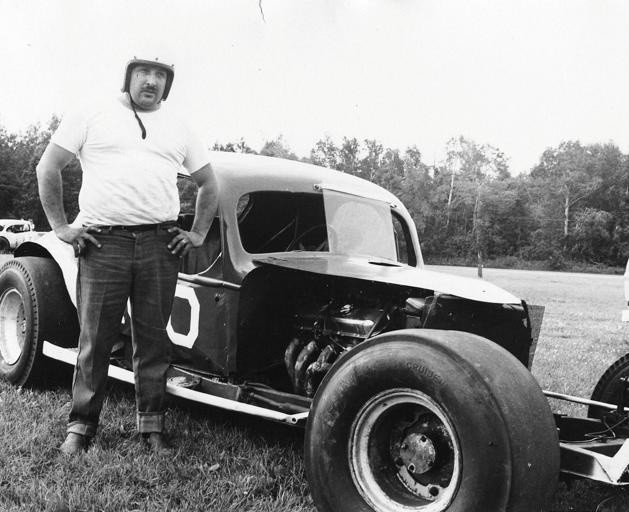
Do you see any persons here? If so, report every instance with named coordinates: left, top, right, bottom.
left=33, top=36, right=225, bottom=459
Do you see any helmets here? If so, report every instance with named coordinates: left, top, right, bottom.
left=120, top=55, right=175, bottom=101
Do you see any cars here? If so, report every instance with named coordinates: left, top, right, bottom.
left=0, top=153, right=629, bottom=512
left=1, top=219, right=39, bottom=252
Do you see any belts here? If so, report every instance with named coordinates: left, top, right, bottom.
left=96, top=221, right=176, bottom=231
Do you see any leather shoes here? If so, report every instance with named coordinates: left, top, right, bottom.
left=142, top=432, right=171, bottom=451
left=59, top=432, right=86, bottom=453
